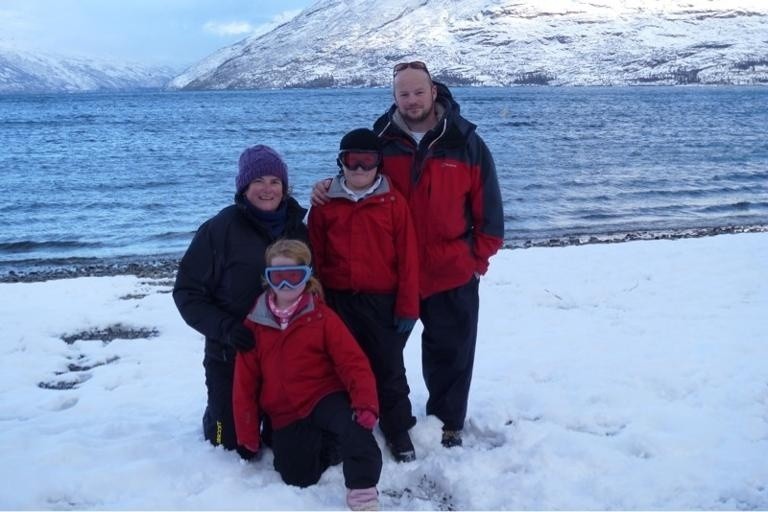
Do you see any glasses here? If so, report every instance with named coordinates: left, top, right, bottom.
left=265, top=263, right=313, bottom=289
left=392, top=61, right=426, bottom=71
left=338, top=149, right=382, bottom=171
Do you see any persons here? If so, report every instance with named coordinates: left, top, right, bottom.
left=172, top=144, right=310, bottom=451
left=232, top=239, right=383, bottom=511
left=307, top=128, right=422, bottom=463
left=309, top=61, right=504, bottom=447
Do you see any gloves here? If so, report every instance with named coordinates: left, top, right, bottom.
left=222, top=323, right=256, bottom=353
left=355, top=409, right=377, bottom=428
left=394, top=316, right=416, bottom=335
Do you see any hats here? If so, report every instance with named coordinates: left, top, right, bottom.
left=337, top=128, right=384, bottom=174
left=236, top=143, right=288, bottom=197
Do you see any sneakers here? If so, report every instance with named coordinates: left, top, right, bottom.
left=347, top=485, right=380, bottom=510
left=441, top=428, right=462, bottom=447
left=390, top=430, right=416, bottom=461
